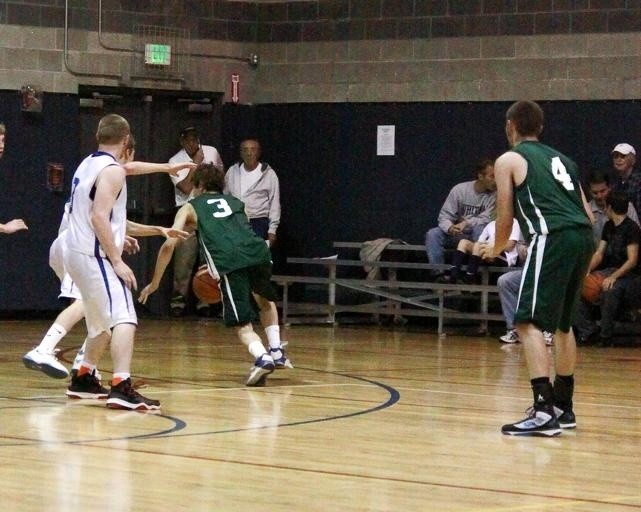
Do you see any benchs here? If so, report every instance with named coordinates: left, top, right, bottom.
left=262, top=235, right=527, bottom=339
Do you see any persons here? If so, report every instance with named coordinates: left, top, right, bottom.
left=425, top=161, right=497, bottom=284
left=477, top=95, right=598, bottom=436
left=573, top=189, right=641, bottom=348
left=0, top=120, right=30, bottom=235
left=166, top=124, right=229, bottom=319
left=497, top=242, right=554, bottom=345
left=439, top=219, right=524, bottom=284
left=588, top=169, right=640, bottom=250
left=223, top=134, right=284, bottom=252
left=612, top=143, right=640, bottom=221
left=138, top=161, right=294, bottom=386
left=63, top=112, right=161, bottom=410
left=22, top=131, right=199, bottom=383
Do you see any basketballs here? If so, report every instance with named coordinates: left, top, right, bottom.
left=581, top=271, right=607, bottom=302
left=193, top=266, right=220, bottom=305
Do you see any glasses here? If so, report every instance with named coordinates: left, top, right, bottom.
left=614, top=154, right=627, bottom=158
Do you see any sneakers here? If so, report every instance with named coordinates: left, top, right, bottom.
left=170, top=297, right=185, bottom=317
left=462, top=272, right=477, bottom=284
left=500, top=330, right=517, bottom=343
left=198, top=302, right=211, bottom=317
left=269, top=342, right=293, bottom=369
left=246, top=353, right=275, bottom=386
left=597, top=330, right=617, bottom=345
left=543, top=331, right=556, bottom=346
left=580, top=333, right=600, bottom=348
left=524, top=404, right=576, bottom=429
left=23, top=346, right=160, bottom=412
left=437, top=271, right=457, bottom=285
left=502, top=403, right=561, bottom=436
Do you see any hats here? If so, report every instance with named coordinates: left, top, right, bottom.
left=611, top=143, right=636, bottom=155
